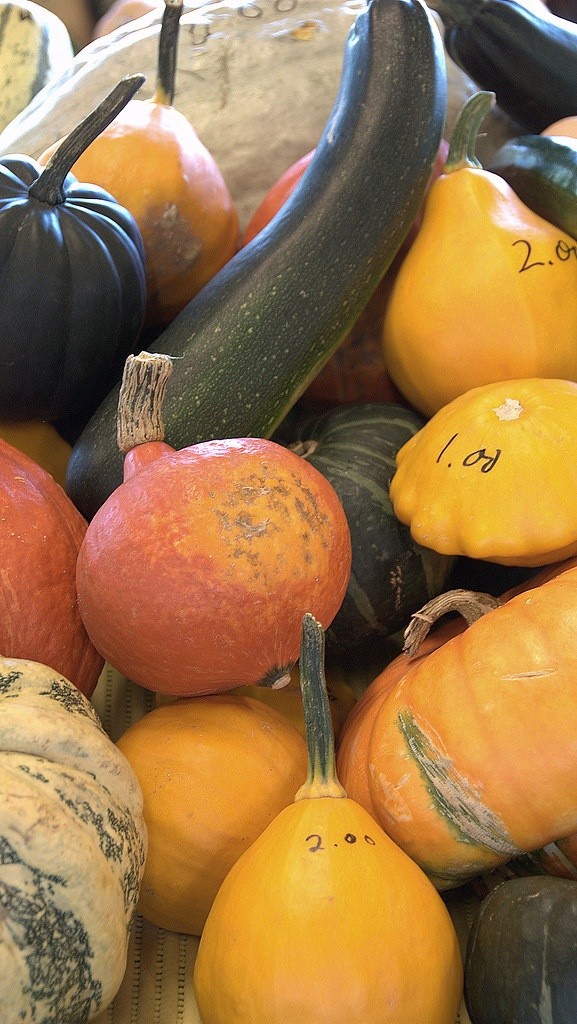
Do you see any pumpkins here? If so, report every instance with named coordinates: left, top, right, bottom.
left=0, top=0, right=577, bottom=1024
